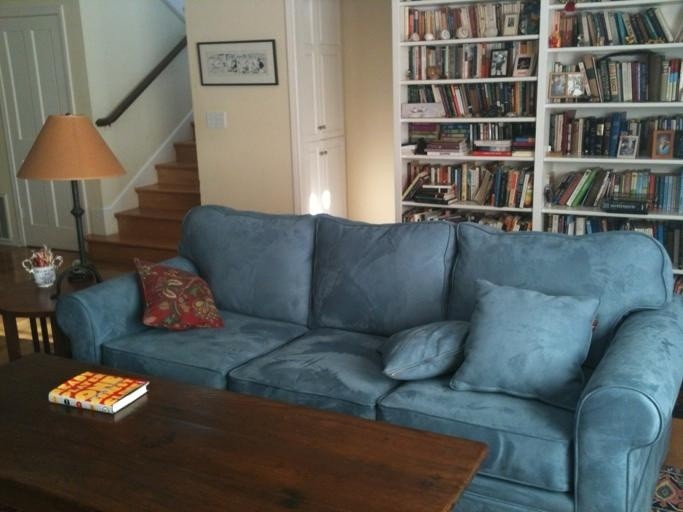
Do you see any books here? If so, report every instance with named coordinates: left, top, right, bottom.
left=48, top=371, right=150, bottom=414
left=48, top=394, right=149, bottom=423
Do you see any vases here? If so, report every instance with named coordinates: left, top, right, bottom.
left=19, top=256, right=62, bottom=287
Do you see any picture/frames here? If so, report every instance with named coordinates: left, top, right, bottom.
left=193, top=39, right=278, bottom=87
left=489, top=48, right=510, bottom=78
left=651, top=129, right=674, bottom=159
left=499, top=11, right=521, bottom=37
left=548, top=71, right=584, bottom=103
left=616, top=134, right=639, bottom=159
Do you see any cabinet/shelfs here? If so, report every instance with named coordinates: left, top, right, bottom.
left=545, top=2, right=682, bottom=274
left=285, top=0, right=346, bottom=220
left=393, top=1, right=541, bottom=234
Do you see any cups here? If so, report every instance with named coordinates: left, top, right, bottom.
left=31, top=266, right=55, bottom=289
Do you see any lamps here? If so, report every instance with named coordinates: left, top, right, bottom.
left=16, top=112, right=129, bottom=288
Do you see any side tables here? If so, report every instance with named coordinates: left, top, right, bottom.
left=0, top=254, right=100, bottom=366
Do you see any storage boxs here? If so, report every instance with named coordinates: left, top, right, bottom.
left=399, top=103, right=445, bottom=118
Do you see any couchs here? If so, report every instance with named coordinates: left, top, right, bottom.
left=55, top=204, right=682, bottom=511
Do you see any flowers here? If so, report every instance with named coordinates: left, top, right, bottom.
left=27, top=243, right=55, bottom=268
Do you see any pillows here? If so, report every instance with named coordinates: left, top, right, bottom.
left=446, top=274, right=601, bottom=413
left=377, top=319, right=471, bottom=383
left=132, top=259, right=223, bottom=332
